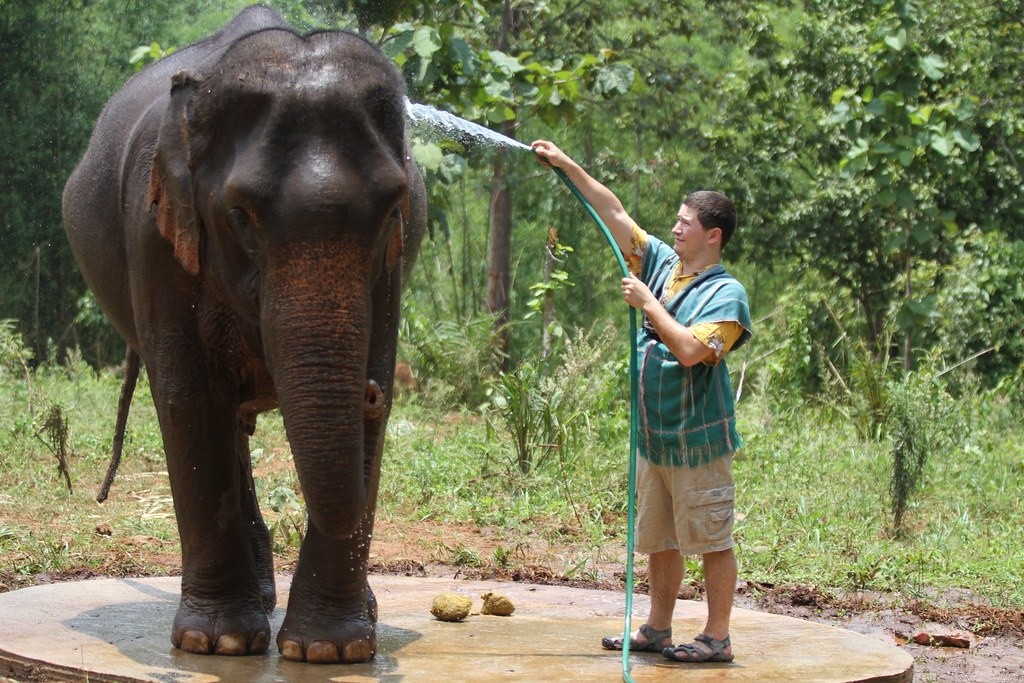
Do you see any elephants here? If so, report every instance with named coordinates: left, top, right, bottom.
left=61, top=2, right=429, bottom=666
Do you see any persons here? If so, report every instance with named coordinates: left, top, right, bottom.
left=531, top=139, right=753, bottom=663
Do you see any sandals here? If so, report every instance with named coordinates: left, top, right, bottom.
left=602, top=624, right=675, bottom=652
left=663, top=634, right=735, bottom=662
